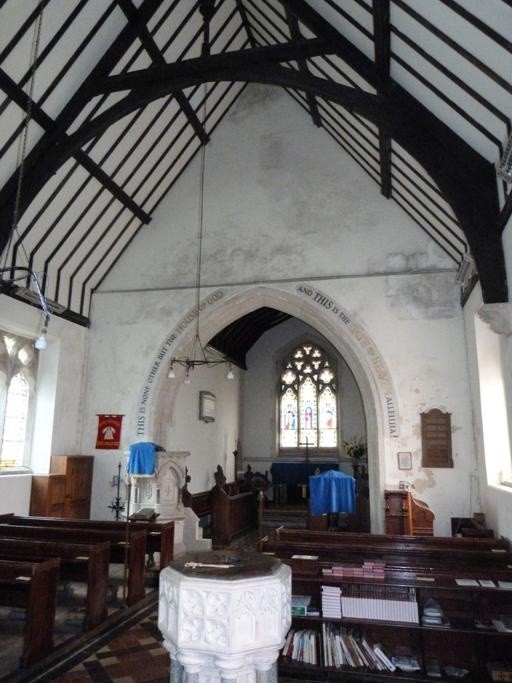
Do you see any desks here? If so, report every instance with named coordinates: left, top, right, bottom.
left=158, top=547, right=294, bottom=683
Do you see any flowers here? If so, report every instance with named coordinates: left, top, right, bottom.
left=345, top=438, right=365, bottom=458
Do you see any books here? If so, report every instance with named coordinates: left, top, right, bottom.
left=280, top=553, right=511, bottom=683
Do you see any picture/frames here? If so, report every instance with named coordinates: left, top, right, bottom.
left=398, top=452, right=412, bottom=470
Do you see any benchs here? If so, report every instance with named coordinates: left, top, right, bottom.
left=451, top=517, right=493, bottom=537
left=1, top=512, right=175, bottom=670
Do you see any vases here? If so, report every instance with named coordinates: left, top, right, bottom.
left=351, top=456, right=359, bottom=466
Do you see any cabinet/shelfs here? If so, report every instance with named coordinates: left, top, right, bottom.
left=51, top=455, right=94, bottom=519
left=257, top=526, right=512, bottom=682
left=272, top=462, right=339, bottom=505
left=30, top=475, right=68, bottom=517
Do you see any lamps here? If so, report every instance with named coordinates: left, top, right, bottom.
left=168, top=82, right=234, bottom=385
left=1, top=11, right=52, bottom=351
